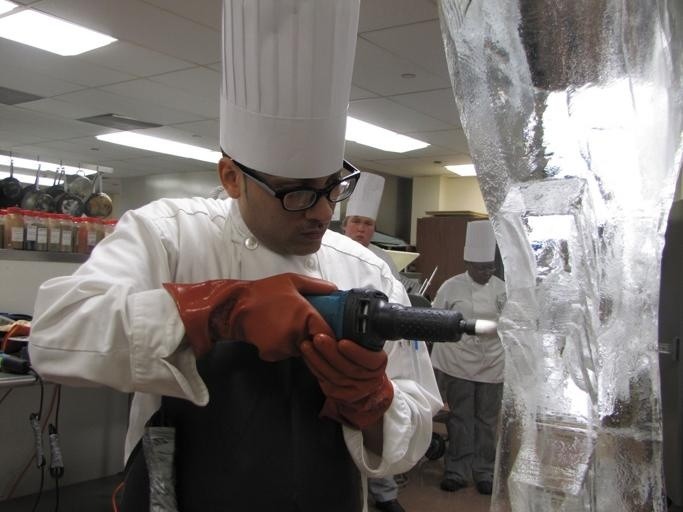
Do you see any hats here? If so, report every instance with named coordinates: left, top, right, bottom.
left=346, top=172, right=385, bottom=221
left=464, top=220, right=496, bottom=263
left=220, top=0, right=360, bottom=179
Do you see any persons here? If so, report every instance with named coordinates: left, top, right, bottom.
left=27, top=147, right=445, bottom=509
left=429, top=260, right=507, bottom=495
left=339, top=215, right=407, bottom=511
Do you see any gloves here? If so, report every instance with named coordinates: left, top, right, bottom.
left=163, top=272, right=336, bottom=363
left=297, top=333, right=394, bottom=431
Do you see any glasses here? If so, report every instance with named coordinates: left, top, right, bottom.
left=230, top=159, right=361, bottom=212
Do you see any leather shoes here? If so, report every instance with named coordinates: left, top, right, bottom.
left=375, top=498, right=406, bottom=511
left=440, top=479, right=494, bottom=493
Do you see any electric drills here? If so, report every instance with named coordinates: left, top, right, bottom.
left=306, top=283, right=567, bottom=352
